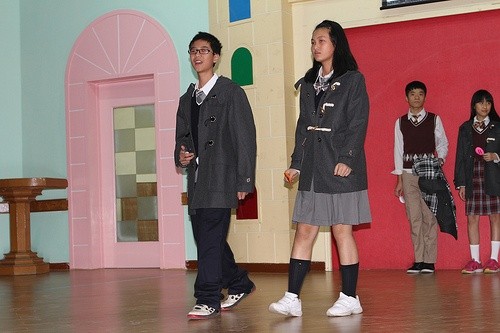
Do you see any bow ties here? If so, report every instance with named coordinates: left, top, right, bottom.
left=474, top=121, right=485, bottom=129
left=313, top=76, right=329, bottom=95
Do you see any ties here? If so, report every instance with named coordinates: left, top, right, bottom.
left=412, top=114, right=421, bottom=125
left=196, top=91, right=206, bottom=103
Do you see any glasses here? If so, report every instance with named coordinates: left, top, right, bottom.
left=188, top=48, right=215, bottom=55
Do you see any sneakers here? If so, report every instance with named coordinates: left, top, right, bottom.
left=327, top=291, right=363, bottom=317
left=484, top=259, right=500, bottom=273
left=221, top=284, right=256, bottom=311
left=406, top=262, right=424, bottom=273
left=420, top=263, right=435, bottom=273
left=461, top=260, right=483, bottom=274
left=269, top=291, right=302, bottom=317
left=187, top=303, right=221, bottom=320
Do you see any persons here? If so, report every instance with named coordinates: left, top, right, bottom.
left=391, top=81, right=448, bottom=273
left=453, top=89, right=500, bottom=274
left=174, top=32, right=257, bottom=320
left=269, top=20, right=372, bottom=317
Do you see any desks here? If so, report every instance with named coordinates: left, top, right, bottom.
left=0, top=177, right=68, bottom=276
